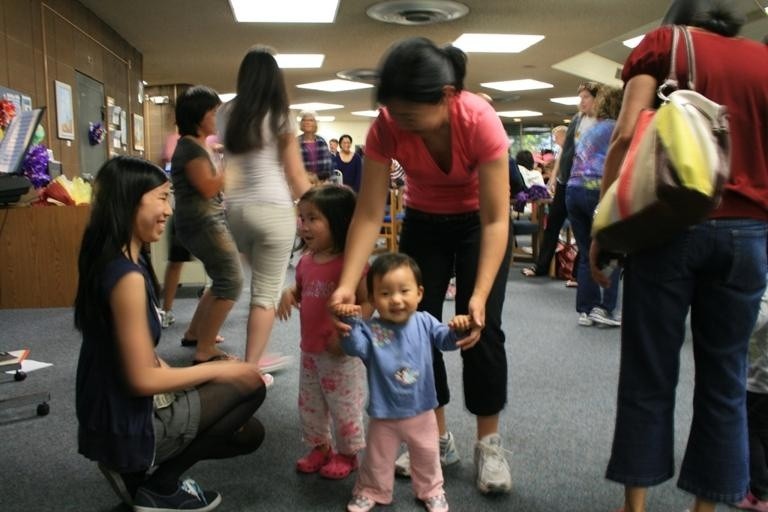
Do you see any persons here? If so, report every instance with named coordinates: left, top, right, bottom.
left=322, top=34, right=513, bottom=497
left=587, top=1, right=766, bottom=511
left=158, top=127, right=222, bottom=328
left=293, top=113, right=330, bottom=176
left=570, top=90, right=626, bottom=329
left=73, top=158, right=268, bottom=509
left=386, top=160, right=407, bottom=206
left=212, top=46, right=317, bottom=386
left=737, top=291, right=766, bottom=510
left=171, top=86, right=243, bottom=368
left=275, top=184, right=374, bottom=479
left=329, top=135, right=363, bottom=190
left=477, top=84, right=600, bottom=287
left=332, top=255, right=475, bottom=510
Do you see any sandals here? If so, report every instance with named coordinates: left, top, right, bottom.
left=294, top=442, right=360, bottom=480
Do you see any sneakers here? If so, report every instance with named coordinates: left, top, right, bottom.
left=347, top=492, right=376, bottom=512
left=577, top=305, right=622, bottom=328
left=161, top=310, right=176, bottom=330
left=256, top=355, right=294, bottom=387
left=472, top=431, right=513, bottom=495
left=394, top=431, right=461, bottom=477
left=423, top=494, right=449, bottom=512
left=520, top=245, right=534, bottom=256
left=92, top=456, right=223, bottom=512
left=734, top=490, right=768, bottom=511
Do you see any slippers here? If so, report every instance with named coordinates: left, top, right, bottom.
left=564, top=275, right=581, bottom=287
left=522, top=265, right=537, bottom=278
left=179, top=334, right=241, bottom=365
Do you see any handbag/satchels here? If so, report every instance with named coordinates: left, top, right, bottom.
left=589, top=22, right=732, bottom=262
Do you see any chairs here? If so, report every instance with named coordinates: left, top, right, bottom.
left=374, top=181, right=565, bottom=282
